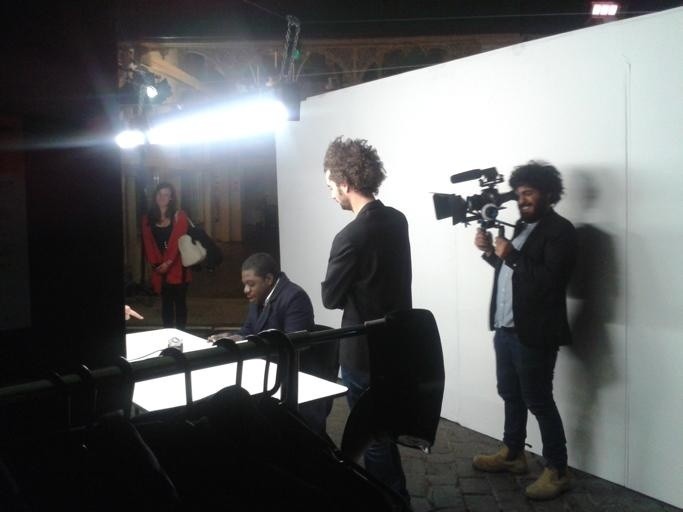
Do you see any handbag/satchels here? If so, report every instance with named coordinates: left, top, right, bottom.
left=178, top=234, right=207, bottom=267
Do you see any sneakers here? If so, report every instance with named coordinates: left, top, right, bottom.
left=521, top=466, right=576, bottom=500
left=472, top=444, right=528, bottom=474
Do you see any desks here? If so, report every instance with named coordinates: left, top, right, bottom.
left=121, top=325, right=347, bottom=429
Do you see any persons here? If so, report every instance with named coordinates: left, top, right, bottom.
left=319, top=135, right=413, bottom=509
left=206, top=253, right=315, bottom=370
left=470, top=161, right=577, bottom=503
left=123, top=302, right=144, bottom=323
left=142, top=179, right=192, bottom=333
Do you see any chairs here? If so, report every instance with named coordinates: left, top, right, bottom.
left=304, top=325, right=342, bottom=436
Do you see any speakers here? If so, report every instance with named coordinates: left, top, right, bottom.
left=24, top=141, right=126, bottom=360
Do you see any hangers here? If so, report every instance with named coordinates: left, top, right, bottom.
left=49, top=323, right=299, bottom=463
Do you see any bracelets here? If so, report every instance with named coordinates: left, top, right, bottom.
left=166, top=260, right=172, bottom=267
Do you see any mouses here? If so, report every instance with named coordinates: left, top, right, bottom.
left=159, top=348, right=181, bottom=356
